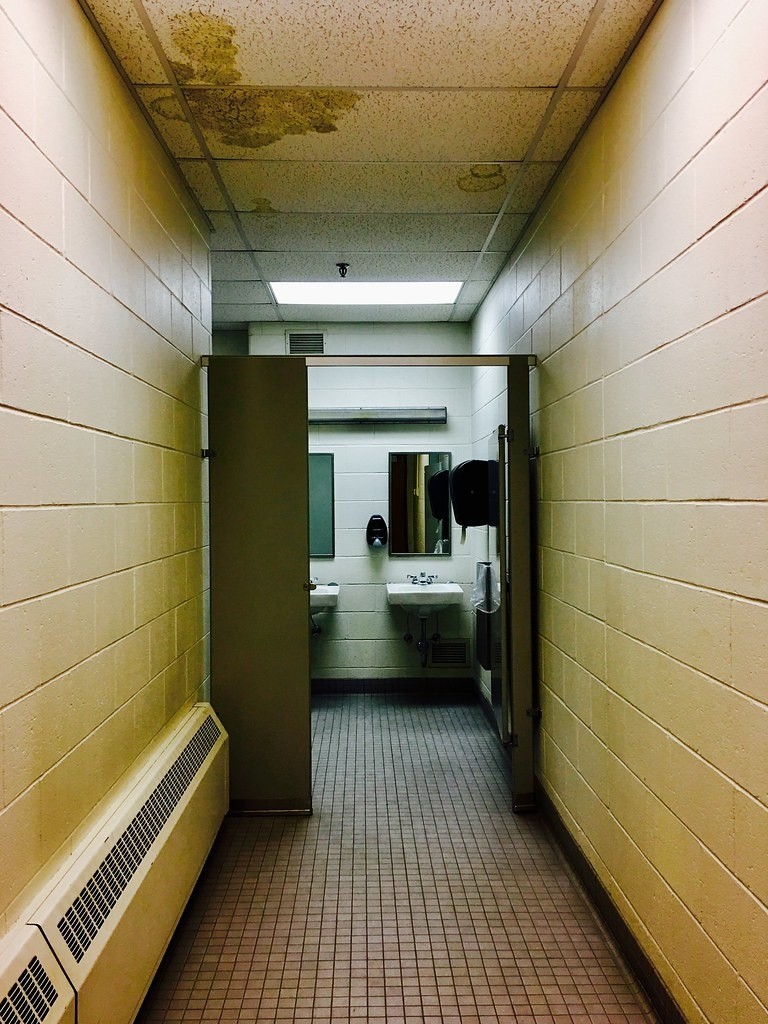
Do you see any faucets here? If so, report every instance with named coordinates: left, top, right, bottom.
left=419, top=571, right=427, bottom=584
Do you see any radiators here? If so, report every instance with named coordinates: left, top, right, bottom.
left=0, top=700, right=231, bottom=1021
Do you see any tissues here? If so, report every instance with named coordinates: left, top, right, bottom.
left=448, top=458, right=500, bottom=546
left=425, top=469, right=449, bottom=535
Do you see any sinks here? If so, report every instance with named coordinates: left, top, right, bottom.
left=386, top=582, right=464, bottom=618
left=309, top=585, right=340, bottom=616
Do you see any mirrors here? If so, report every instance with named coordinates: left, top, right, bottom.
left=389, top=450, right=452, bottom=557
left=308, top=452, right=336, bottom=558
